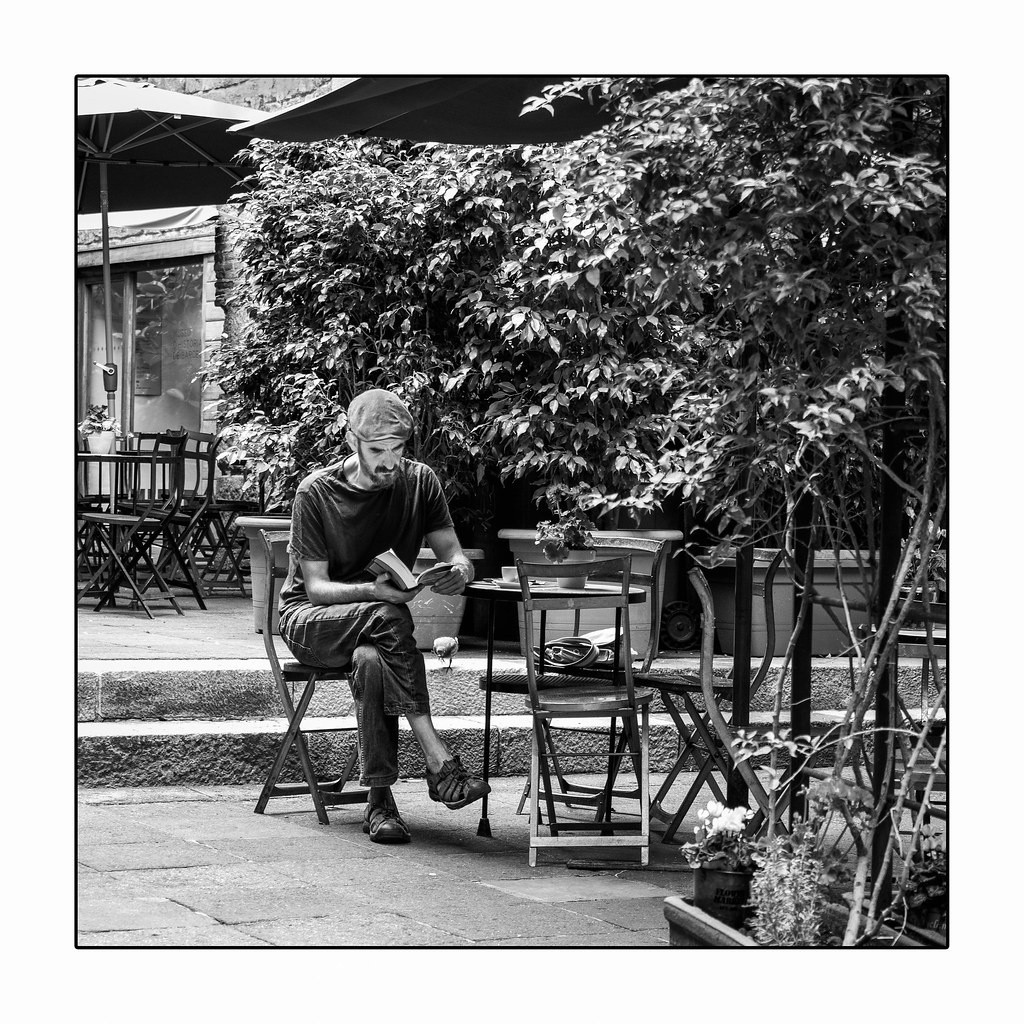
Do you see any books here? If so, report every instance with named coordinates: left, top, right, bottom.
left=365, top=548, right=456, bottom=592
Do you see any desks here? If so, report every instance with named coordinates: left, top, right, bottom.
left=856, top=623, right=949, bottom=843
left=460, top=580, right=647, bottom=837
left=77, top=452, right=181, bottom=608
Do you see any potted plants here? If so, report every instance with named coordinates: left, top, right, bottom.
left=498, top=529, right=684, bottom=661
left=403, top=548, right=485, bottom=651
left=77, top=403, right=120, bottom=454
left=214, top=420, right=301, bottom=635
left=534, top=508, right=598, bottom=589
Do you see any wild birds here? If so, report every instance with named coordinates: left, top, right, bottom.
left=432, top=636, right=459, bottom=676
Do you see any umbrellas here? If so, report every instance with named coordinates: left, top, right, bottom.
left=222, top=75, right=730, bottom=152
left=74, top=75, right=337, bottom=608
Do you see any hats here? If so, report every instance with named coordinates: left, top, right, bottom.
left=348, top=388, right=414, bottom=443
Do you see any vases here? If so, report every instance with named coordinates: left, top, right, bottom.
left=694, top=868, right=758, bottom=929
left=664, top=895, right=925, bottom=947
left=702, top=547, right=880, bottom=657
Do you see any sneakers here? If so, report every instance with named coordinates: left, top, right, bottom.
left=360, top=793, right=410, bottom=842
left=425, top=755, right=492, bottom=810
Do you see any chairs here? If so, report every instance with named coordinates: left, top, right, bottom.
left=630, top=547, right=789, bottom=844
left=254, top=529, right=370, bottom=826
left=75, top=425, right=265, bottom=619
left=516, top=531, right=670, bottom=823
left=515, top=554, right=653, bottom=867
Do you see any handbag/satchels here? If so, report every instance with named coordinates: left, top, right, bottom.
left=531, top=626, right=637, bottom=670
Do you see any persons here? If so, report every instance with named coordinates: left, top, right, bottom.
left=276, top=385, right=490, bottom=842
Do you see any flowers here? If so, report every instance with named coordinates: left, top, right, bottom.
left=678, top=800, right=755, bottom=869
